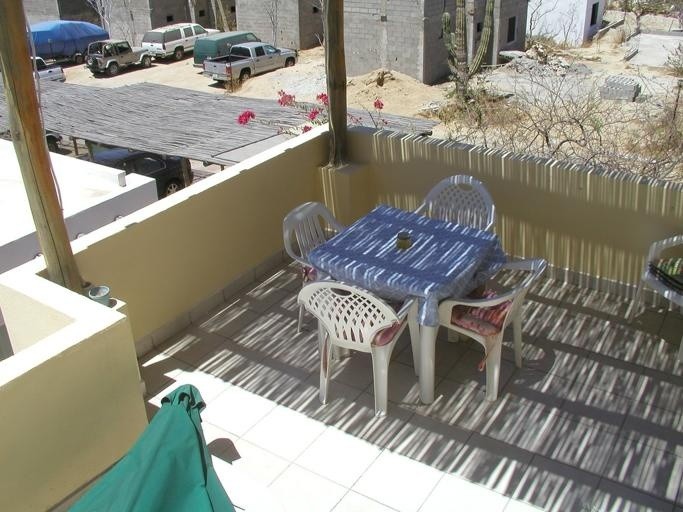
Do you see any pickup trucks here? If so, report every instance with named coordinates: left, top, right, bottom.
left=204, top=42, right=297, bottom=84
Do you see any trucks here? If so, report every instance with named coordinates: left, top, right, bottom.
left=29, top=56, right=66, bottom=84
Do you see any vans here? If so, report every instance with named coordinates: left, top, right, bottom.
left=24, top=20, right=110, bottom=64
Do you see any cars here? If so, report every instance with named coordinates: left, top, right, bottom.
left=80, top=149, right=193, bottom=199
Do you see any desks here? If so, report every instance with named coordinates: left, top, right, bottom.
left=310, top=205, right=490, bottom=405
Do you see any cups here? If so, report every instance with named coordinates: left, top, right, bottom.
left=88, top=285, right=110, bottom=306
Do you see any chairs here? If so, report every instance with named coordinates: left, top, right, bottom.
left=298, top=281, right=422, bottom=419
left=625, top=237, right=683, bottom=363
left=439, top=258, right=547, bottom=404
left=283, top=201, right=344, bottom=332
left=414, top=176, right=496, bottom=338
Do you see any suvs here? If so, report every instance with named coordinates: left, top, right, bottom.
left=87, top=40, right=151, bottom=76
left=191, top=31, right=261, bottom=68
left=143, top=23, right=220, bottom=61
